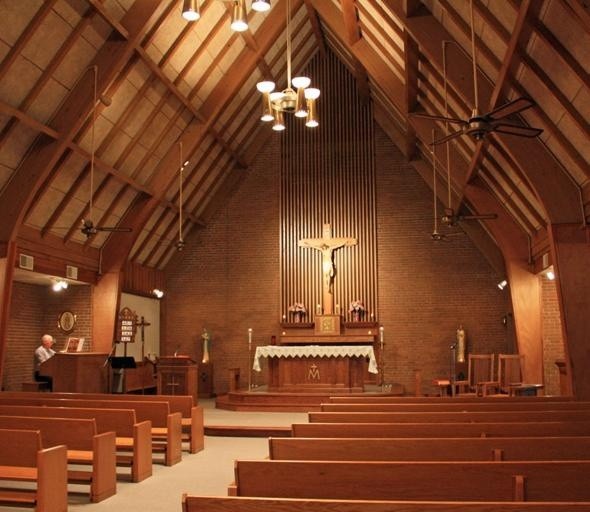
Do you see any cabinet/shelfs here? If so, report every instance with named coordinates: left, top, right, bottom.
left=159, top=365, right=197, bottom=406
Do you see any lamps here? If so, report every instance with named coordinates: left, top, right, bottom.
left=256, top=0, right=321, bottom=130
left=183, top=0, right=271, bottom=31
left=498, top=280, right=507, bottom=290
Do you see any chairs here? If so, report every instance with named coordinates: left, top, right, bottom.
left=455, top=353, right=495, bottom=397
left=484, top=354, right=526, bottom=397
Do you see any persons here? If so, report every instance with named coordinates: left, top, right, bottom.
left=299, top=240, right=354, bottom=292
left=456, top=324, right=466, bottom=363
left=74, top=340, right=79, bottom=351
left=70, top=340, right=74, bottom=351
left=34, top=334, right=56, bottom=392
left=201, top=327, right=210, bottom=363
left=64, top=312, right=71, bottom=328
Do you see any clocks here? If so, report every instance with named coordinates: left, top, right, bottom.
left=58, top=310, right=77, bottom=334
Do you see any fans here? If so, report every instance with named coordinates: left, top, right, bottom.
left=430, top=128, right=464, bottom=242
left=177, top=140, right=187, bottom=251
left=442, top=42, right=497, bottom=235
left=46, top=66, right=132, bottom=233
left=408, top=0, right=543, bottom=145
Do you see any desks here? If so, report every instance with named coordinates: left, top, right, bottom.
left=252, top=345, right=378, bottom=392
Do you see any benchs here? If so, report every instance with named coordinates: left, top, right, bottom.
left=308, top=409, right=590, bottom=423
left=1, top=428, right=68, bottom=512
left=0, top=415, right=117, bottom=502
left=181, top=493, right=590, bottom=512
left=227, top=459, right=590, bottom=502
left=0, top=404, right=152, bottom=482
left=23, top=381, right=48, bottom=391
left=292, top=421, right=590, bottom=438
left=0, top=390, right=204, bottom=454
left=330, top=397, right=590, bottom=403
left=269, top=436, right=590, bottom=462
left=321, top=403, right=590, bottom=412
left=0, top=396, right=181, bottom=466
